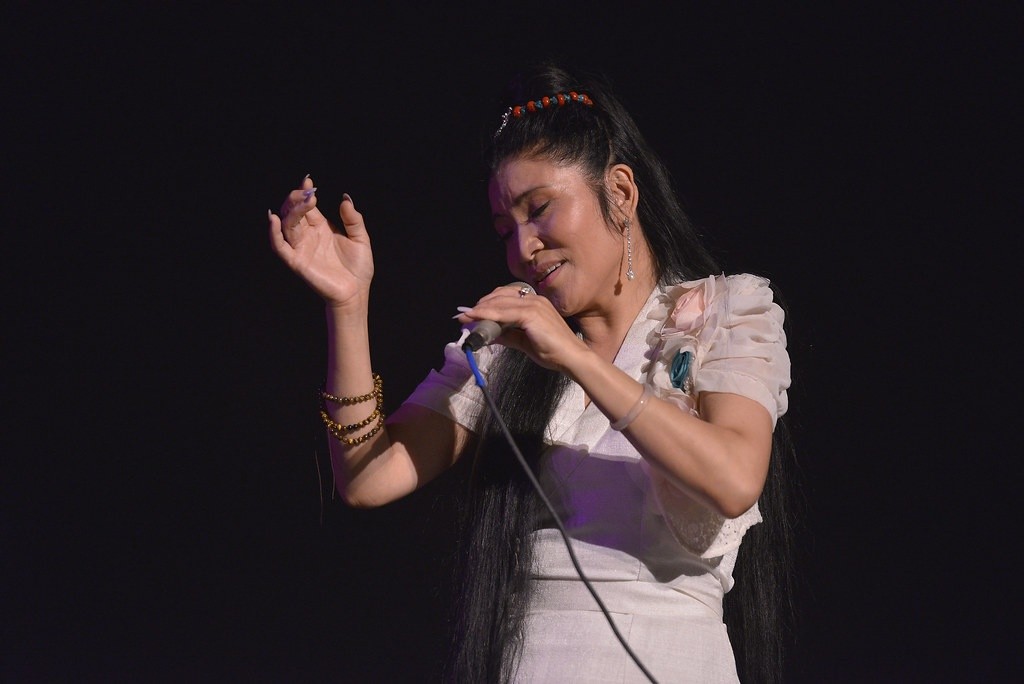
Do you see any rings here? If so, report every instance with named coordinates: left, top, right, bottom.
left=519, top=284, right=530, bottom=296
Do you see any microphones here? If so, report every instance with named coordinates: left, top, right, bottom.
left=461, top=281, right=537, bottom=352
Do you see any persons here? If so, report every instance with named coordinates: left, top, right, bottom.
left=267, top=56, right=801, bottom=684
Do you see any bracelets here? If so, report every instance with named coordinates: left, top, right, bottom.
left=611, top=382, right=650, bottom=430
left=318, top=374, right=385, bottom=443
